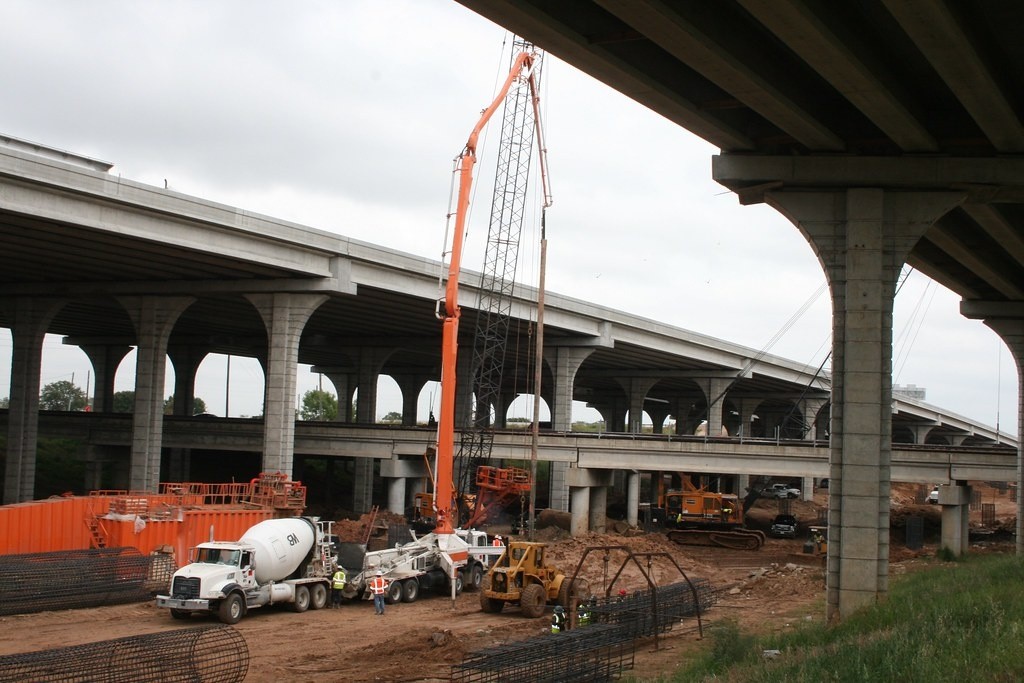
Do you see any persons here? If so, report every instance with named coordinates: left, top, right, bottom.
left=551, top=605, right=567, bottom=634
left=578, top=595, right=598, bottom=628
left=370, top=571, right=388, bottom=615
left=492, top=535, right=504, bottom=564
left=616, top=590, right=628, bottom=603
left=721, top=504, right=729, bottom=522
left=332, top=565, right=346, bottom=609
left=813, top=531, right=824, bottom=551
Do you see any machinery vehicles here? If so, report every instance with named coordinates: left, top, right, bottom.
left=657, top=470, right=765, bottom=550
left=336, top=33, right=589, bottom=618
left=156, top=515, right=331, bottom=625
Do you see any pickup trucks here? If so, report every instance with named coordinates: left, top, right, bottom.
left=929, top=485, right=938, bottom=503
left=760, top=483, right=800, bottom=500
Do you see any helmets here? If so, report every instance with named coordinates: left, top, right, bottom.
left=498, top=536, right=502, bottom=539
left=554, top=606, right=564, bottom=613
left=495, top=534, right=498, bottom=538
left=589, top=596, right=597, bottom=601
left=376, top=571, right=382, bottom=575
left=579, top=605, right=585, bottom=610
left=337, top=565, right=342, bottom=569
left=618, top=589, right=626, bottom=595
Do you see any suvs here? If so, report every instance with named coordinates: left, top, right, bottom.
left=770, top=515, right=800, bottom=538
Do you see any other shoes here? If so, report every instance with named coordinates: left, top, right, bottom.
left=375, top=612, right=379, bottom=615
left=379, top=613, right=384, bottom=615
